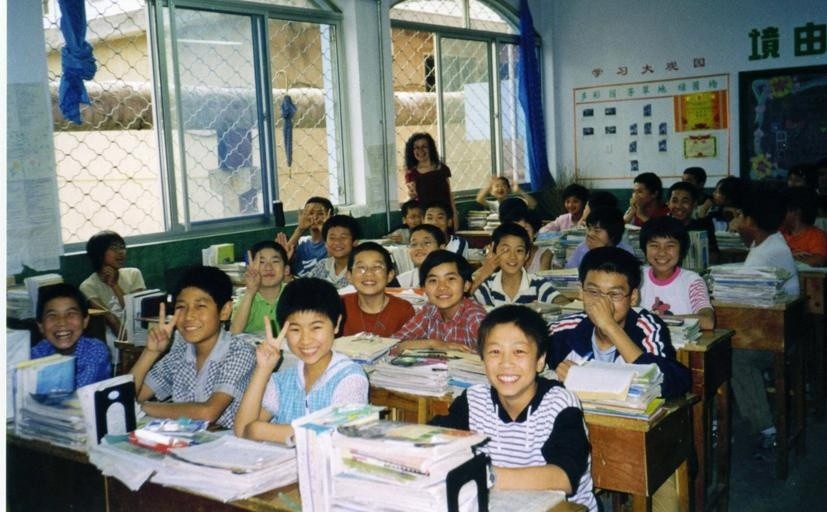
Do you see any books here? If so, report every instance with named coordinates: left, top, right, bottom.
left=6, top=283, right=34, bottom=321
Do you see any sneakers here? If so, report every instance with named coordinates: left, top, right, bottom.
left=713, top=427, right=735, bottom=448
left=745, top=433, right=776, bottom=464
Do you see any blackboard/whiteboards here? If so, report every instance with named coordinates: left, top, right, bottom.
left=738, top=63, right=827, bottom=184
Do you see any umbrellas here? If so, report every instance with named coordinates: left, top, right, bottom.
left=274, top=70, right=296, bottom=178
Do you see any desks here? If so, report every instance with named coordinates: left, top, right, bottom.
left=793, top=254, right=827, bottom=457
left=9, top=308, right=704, bottom=512
left=709, top=292, right=816, bottom=475
left=674, top=323, right=739, bottom=511
left=453, top=231, right=540, bottom=275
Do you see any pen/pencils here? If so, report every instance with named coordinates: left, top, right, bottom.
left=579, top=351, right=593, bottom=365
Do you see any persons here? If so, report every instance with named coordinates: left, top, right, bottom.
left=29, top=134, right=826, bottom=512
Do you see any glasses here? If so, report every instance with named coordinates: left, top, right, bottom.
left=583, top=287, right=629, bottom=301
